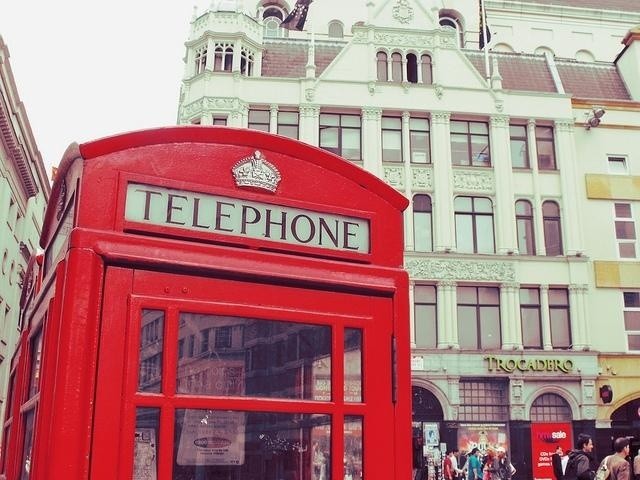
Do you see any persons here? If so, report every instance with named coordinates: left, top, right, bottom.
left=426, top=430, right=437, bottom=443
left=442, top=447, right=516, bottom=480
left=551, top=445, right=563, bottom=480
left=633, top=449, right=640, bottom=479
left=562, top=433, right=596, bottom=480
left=594, top=437, right=630, bottom=480
left=562, top=449, right=573, bottom=474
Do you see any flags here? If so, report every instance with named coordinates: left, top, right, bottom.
left=476, top=7, right=492, bottom=49
left=284, top=3, right=310, bottom=34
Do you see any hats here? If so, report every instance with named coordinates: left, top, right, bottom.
left=495, top=447, right=506, bottom=453
left=615, top=438, right=630, bottom=449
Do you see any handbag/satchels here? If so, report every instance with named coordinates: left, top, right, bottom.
left=594, top=465, right=609, bottom=480
left=510, top=463, right=516, bottom=475
